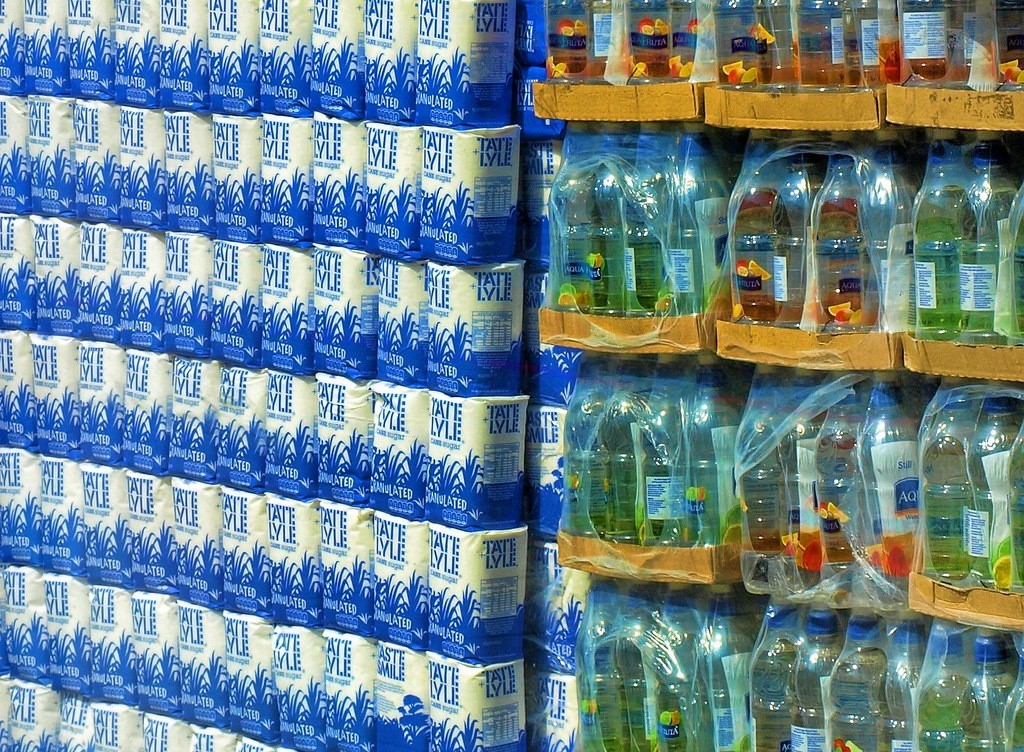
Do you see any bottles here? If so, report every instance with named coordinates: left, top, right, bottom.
left=562, top=354, right=1024, bottom=752
left=551, top=121, right=1024, bottom=345
left=544, top=0, right=1024, bottom=95
